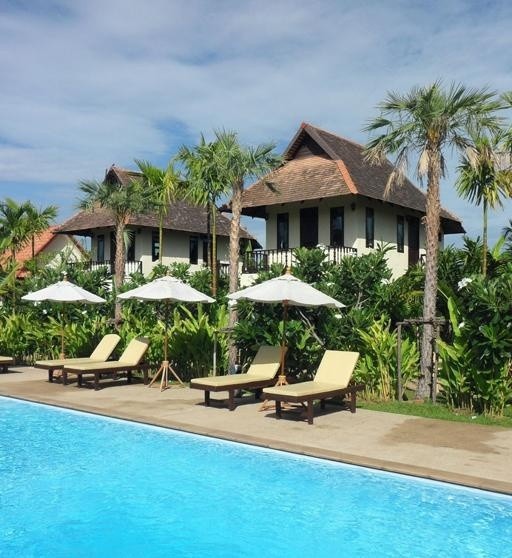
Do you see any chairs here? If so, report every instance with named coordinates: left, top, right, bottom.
left=190, top=344, right=366, bottom=425
left=35, top=333, right=152, bottom=391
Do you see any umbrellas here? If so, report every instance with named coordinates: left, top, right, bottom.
left=20, top=276, right=108, bottom=353
left=115, top=275, right=218, bottom=360
left=225, top=271, right=348, bottom=376
